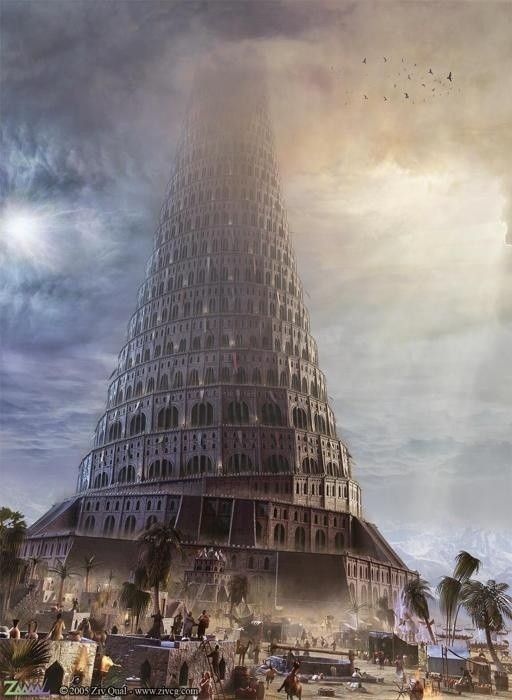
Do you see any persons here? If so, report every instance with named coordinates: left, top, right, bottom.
left=264, top=665, right=277, bottom=690
left=206, top=645, right=222, bottom=676
left=348, top=649, right=404, bottom=688
left=200, top=671, right=213, bottom=700
left=410, top=681, right=423, bottom=700
left=9, top=619, right=21, bottom=639
left=44, top=614, right=66, bottom=641
left=145, top=606, right=210, bottom=640
left=459, top=667, right=472, bottom=686
left=295, top=629, right=336, bottom=656
